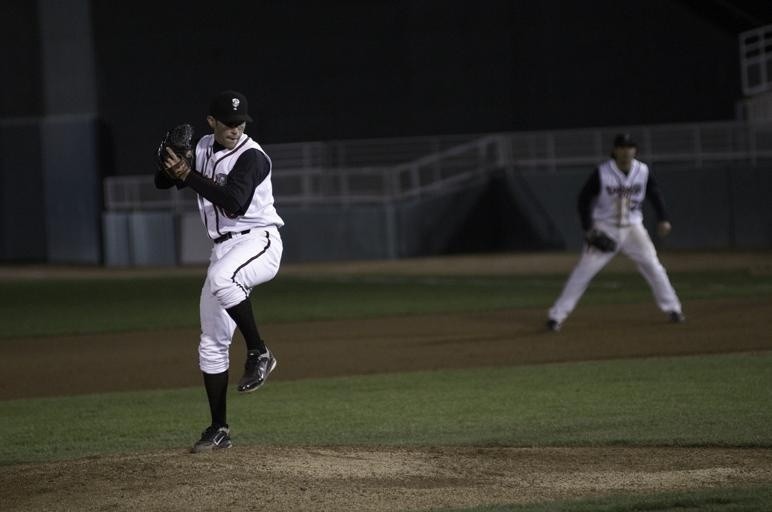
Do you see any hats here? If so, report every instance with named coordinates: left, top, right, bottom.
left=614, top=137, right=637, bottom=146
left=209, top=91, right=253, bottom=123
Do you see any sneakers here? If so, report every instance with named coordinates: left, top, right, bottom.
left=192, top=425, right=232, bottom=453
left=239, top=346, right=277, bottom=393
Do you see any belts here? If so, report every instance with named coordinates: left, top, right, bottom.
left=213, top=229, right=250, bottom=243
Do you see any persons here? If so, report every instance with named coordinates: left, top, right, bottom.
left=544, top=134, right=685, bottom=328
left=155, top=92, right=285, bottom=453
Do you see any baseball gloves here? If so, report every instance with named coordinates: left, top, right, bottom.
left=155, top=124, right=193, bottom=170
left=594, top=231, right=616, bottom=253
